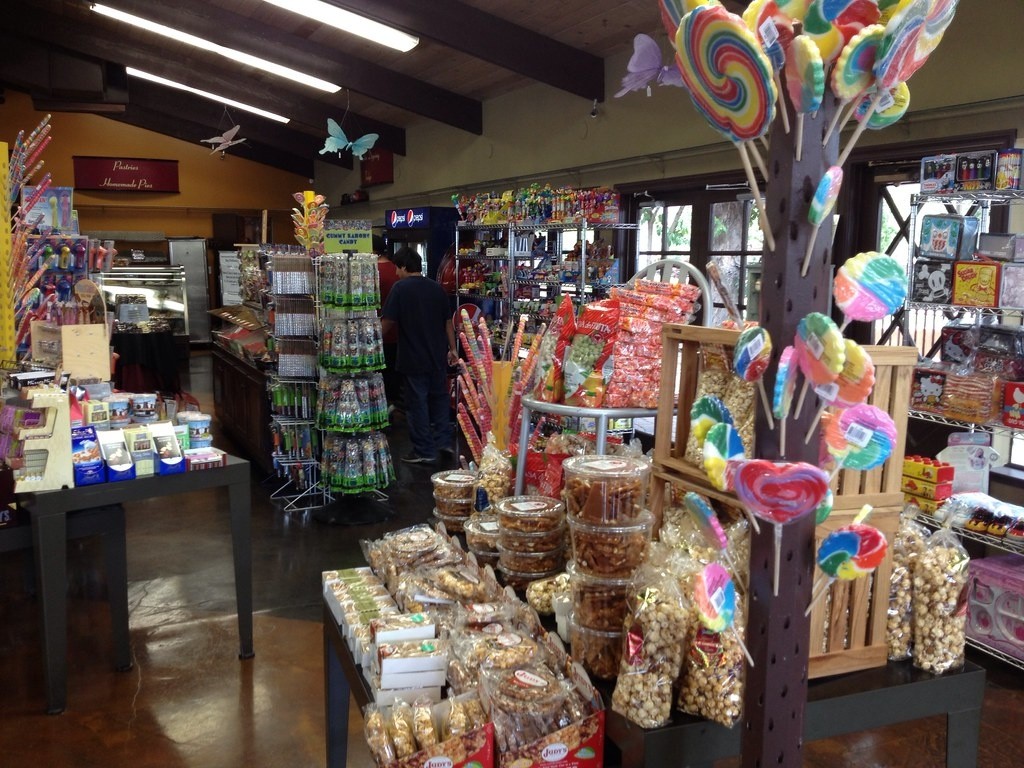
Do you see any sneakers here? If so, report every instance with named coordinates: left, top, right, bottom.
left=438, top=447, right=454, bottom=457
left=400, top=448, right=438, bottom=465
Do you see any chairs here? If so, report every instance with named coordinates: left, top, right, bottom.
left=513, top=259, right=711, bottom=496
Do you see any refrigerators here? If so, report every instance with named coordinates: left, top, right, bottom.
left=383, top=205, right=457, bottom=278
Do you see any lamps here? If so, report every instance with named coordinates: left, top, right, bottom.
left=84, top=0, right=420, bottom=124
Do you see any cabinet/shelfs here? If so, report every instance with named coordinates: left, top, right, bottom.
left=456, top=221, right=640, bottom=455
left=100, top=245, right=395, bottom=525
left=901, top=190, right=1024, bottom=670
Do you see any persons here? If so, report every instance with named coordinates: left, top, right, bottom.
left=380, top=247, right=459, bottom=463
left=372, top=234, right=399, bottom=416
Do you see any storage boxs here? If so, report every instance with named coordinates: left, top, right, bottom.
left=71, top=393, right=228, bottom=486
left=901, top=147, right=1024, bottom=661
left=322, top=521, right=606, bottom=768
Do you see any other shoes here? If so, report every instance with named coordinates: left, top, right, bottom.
left=387, top=404, right=395, bottom=415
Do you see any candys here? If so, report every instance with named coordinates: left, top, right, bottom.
left=455, top=306, right=547, bottom=462
left=655, top=0, right=960, bottom=666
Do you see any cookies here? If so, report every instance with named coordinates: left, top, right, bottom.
left=324, top=444, right=651, bottom=765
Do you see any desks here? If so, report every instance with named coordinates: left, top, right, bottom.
left=324, top=600, right=376, bottom=768
left=0, top=446, right=256, bottom=715
left=427, top=516, right=988, bottom=768
left=0, top=502, right=135, bottom=672
left=110, top=332, right=182, bottom=399
